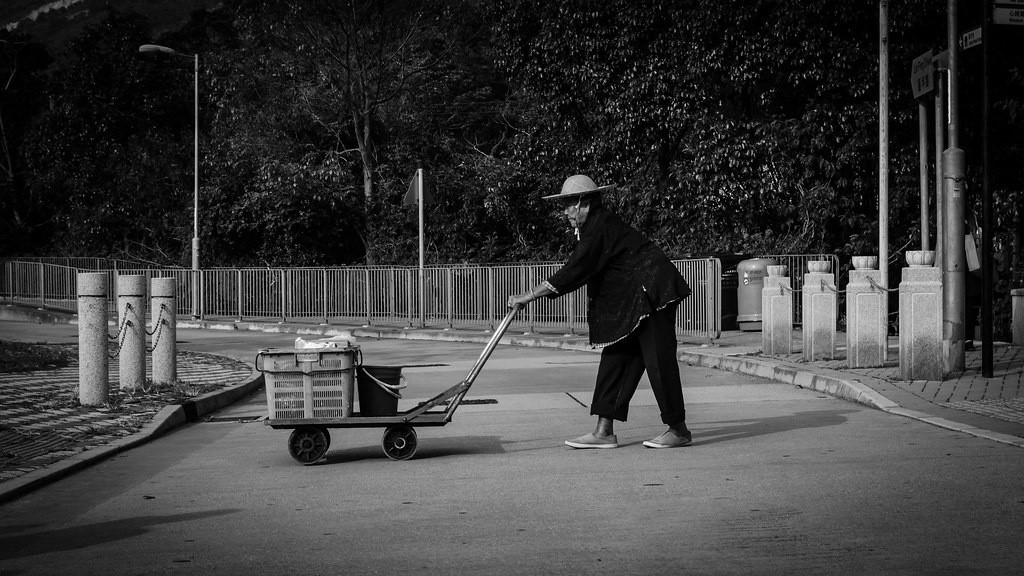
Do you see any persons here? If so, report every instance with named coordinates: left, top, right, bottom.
left=507, top=174, right=693, bottom=449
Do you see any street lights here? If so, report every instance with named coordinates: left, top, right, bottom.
left=138, top=42, right=202, bottom=319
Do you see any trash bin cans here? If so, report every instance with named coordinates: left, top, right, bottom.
left=736, top=258, right=774, bottom=332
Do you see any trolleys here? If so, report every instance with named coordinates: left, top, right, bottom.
left=238, top=301, right=524, bottom=465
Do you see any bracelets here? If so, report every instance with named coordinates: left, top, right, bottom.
left=529, top=287, right=537, bottom=301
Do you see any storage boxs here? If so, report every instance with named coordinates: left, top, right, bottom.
left=257, top=345, right=362, bottom=417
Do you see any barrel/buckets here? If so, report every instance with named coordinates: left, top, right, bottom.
left=355, top=365, right=407, bottom=416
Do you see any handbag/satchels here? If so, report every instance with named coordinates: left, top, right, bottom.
left=965, top=214, right=983, bottom=271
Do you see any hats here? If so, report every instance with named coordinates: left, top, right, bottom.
left=541, top=174, right=617, bottom=199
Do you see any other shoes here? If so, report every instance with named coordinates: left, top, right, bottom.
left=643, top=428, right=692, bottom=448
left=966, top=341, right=976, bottom=352
left=564, top=433, right=619, bottom=448
